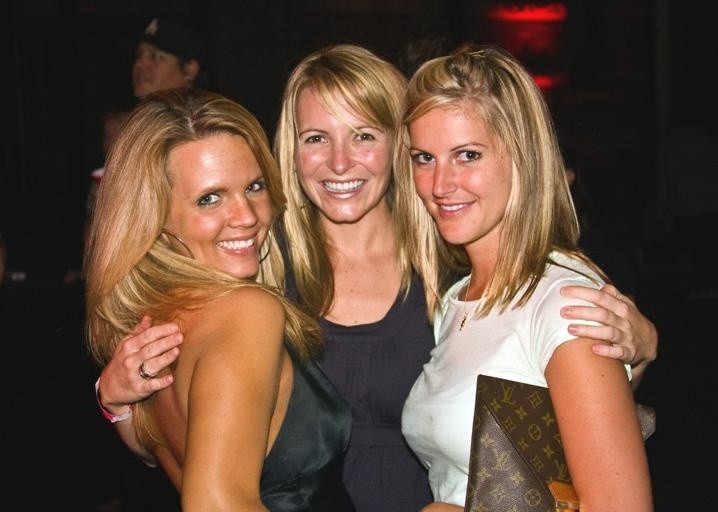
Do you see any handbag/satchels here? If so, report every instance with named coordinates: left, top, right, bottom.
left=464, top=374, right=655, bottom=512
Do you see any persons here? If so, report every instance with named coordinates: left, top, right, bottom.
left=397, top=44, right=661, bottom=510
left=78, top=41, right=660, bottom=511
left=75, top=9, right=208, bottom=207
left=75, top=87, right=362, bottom=512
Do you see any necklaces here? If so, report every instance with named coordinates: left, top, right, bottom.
left=458, top=267, right=496, bottom=330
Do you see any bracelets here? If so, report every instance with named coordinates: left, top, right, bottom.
left=94, top=378, right=135, bottom=423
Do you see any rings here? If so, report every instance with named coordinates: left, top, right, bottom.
left=138, top=362, right=155, bottom=379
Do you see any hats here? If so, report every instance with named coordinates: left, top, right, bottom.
left=143, top=9, right=209, bottom=64
